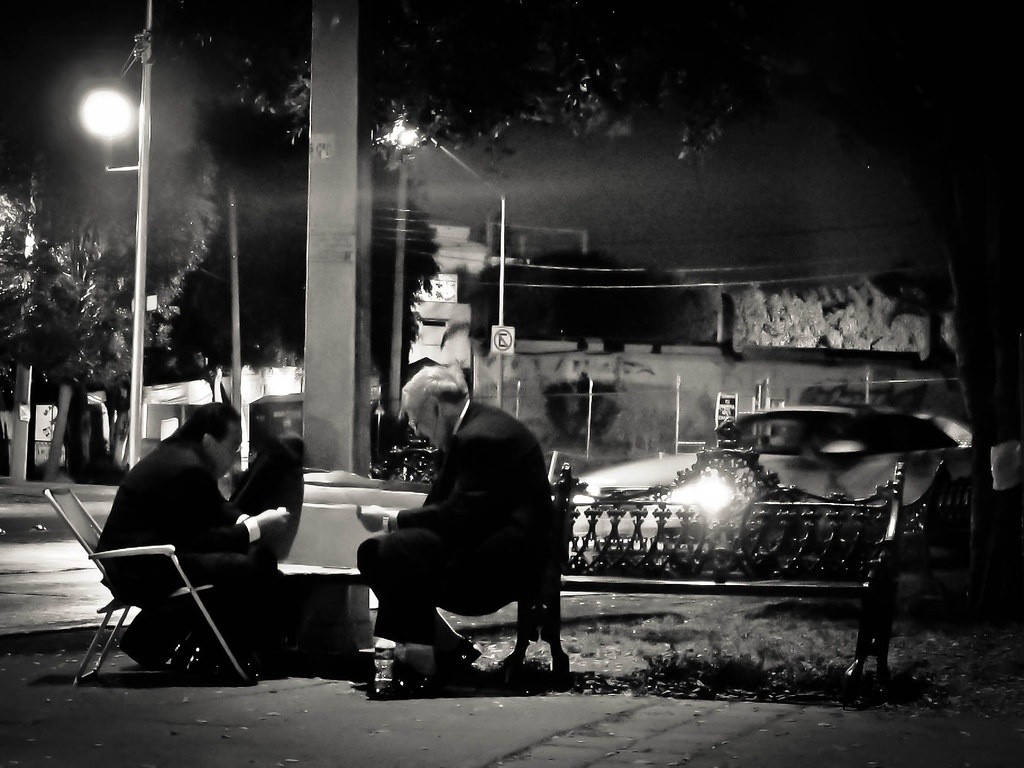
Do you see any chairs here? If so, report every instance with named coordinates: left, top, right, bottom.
left=44, top=486, right=257, bottom=686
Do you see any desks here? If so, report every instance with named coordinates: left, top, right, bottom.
left=274, top=562, right=369, bottom=670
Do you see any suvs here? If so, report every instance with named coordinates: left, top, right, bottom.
left=712, top=402, right=975, bottom=515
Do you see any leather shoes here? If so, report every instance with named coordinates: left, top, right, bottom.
left=365, top=659, right=436, bottom=701
left=444, top=638, right=485, bottom=681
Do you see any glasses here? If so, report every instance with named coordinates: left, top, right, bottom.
left=223, top=443, right=241, bottom=456
left=408, top=397, right=429, bottom=432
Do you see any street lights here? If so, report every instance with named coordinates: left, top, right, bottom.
left=385, top=118, right=506, bottom=410
left=77, top=89, right=153, bottom=467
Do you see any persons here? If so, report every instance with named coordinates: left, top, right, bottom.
left=356, top=366, right=554, bottom=697
left=95, top=403, right=289, bottom=671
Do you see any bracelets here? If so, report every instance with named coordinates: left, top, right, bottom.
left=382, top=513, right=390, bottom=528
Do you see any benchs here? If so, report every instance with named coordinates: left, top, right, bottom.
left=499, top=415, right=907, bottom=708
